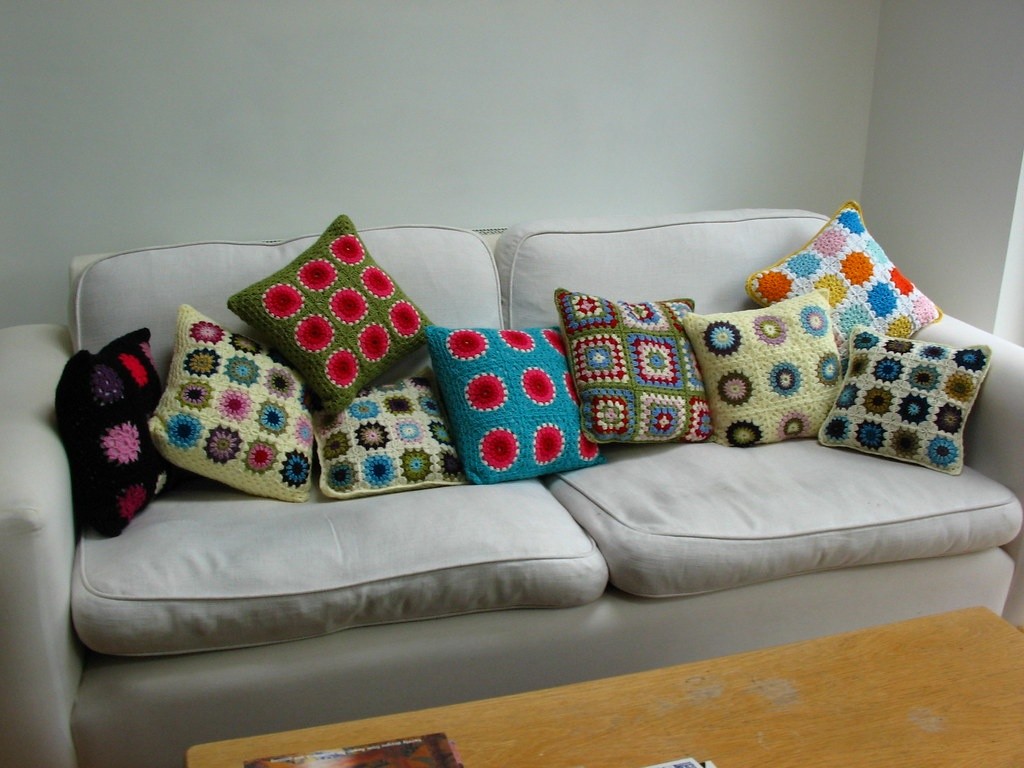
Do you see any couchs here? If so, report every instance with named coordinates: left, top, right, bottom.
left=0, top=208, right=1024, bottom=767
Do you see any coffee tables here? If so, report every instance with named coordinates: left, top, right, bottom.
left=185, top=604, right=1024, bottom=768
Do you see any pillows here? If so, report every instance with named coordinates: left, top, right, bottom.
left=147, top=303, right=315, bottom=503
left=817, top=326, right=992, bottom=476
left=53, top=326, right=180, bottom=536
left=679, top=289, right=848, bottom=448
left=745, top=200, right=944, bottom=375
left=226, top=213, right=437, bottom=419
left=307, top=364, right=468, bottom=498
left=425, top=321, right=606, bottom=485
left=550, top=283, right=712, bottom=444
